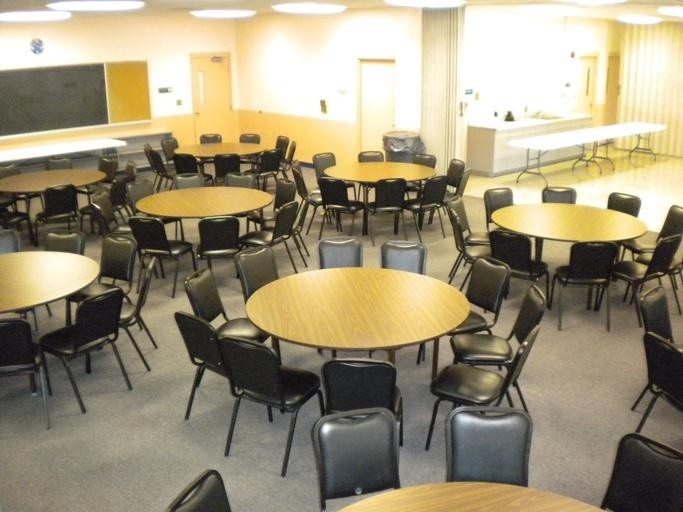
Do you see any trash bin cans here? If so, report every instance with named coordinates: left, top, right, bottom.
left=383, top=130, right=420, bottom=163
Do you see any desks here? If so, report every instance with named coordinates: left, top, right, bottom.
left=238, top=267, right=470, bottom=405
left=325, top=163, right=439, bottom=218
left=0, top=250, right=99, bottom=397
left=333, top=480, right=608, bottom=511
left=487, top=202, right=648, bottom=311
left=506, top=119, right=667, bottom=193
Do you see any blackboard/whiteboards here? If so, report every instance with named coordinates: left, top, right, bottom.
left=0, top=62, right=110, bottom=140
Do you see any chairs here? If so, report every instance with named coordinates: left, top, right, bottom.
left=613, top=234, right=682, bottom=323
left=218, top=334, right=321, bottom=477
left=185, top=268, right=283, bottom=365
left=441, top=404, right=536, bottom=489
left=489, top=230, right=549, bottom=309
left=542, top=185, right=576, bottom=206
left=1, top=228, right=21, bottom=253
left=416, top=256, right=511, bottom=365
left=607, top=192, right=642, bottom=218
left=550, top=242, right=617, bottom=332
left=40, top=287, right=133, bottom=408
left=484, top=187, right=513, bottom=228
left=234, top=246, right=280, bottom=303
left=263, top=196, right=321, bottom=267
left=1, top=314, right=53, bottom=430
left=0, top=130, right=466, bottom=243
left=446, top=198, right=491, bottom=253
left=317, top=237, right=362, bottom=268
left=425, top=327, right=542, bottom=452
left=629, top=332, right=683, bottom=433
left=94, top=257, right=158, bottom=373
left=640, top=285, right=683, bottom=350
left=240, top=201, right=298, bottom=276
left=447, top=209, right=492, bottom=289
left=642, top=205, right=682, bottom=313
left=323, top=359, right=404, bottom=446
left=449, top=285, right=547, bottom=370
left=173, top=311, right=275, bottom=423
left=45, top=231, right=85, bottom=254
left=597, top=430, right=683, bottom=511
left=127, top=213, right=197, bottom=297
left=196, top=216, right=241, bottom=280
left=310, top=406, right=404, bottom=512
left=379, top=241, right=427, bottom=274
left=65, top=234, right=143, bottom=330
left=162, top=468, right=234, bottom=512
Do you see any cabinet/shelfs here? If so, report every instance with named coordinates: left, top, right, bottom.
left=468, top=113, right=592, bottom=179
